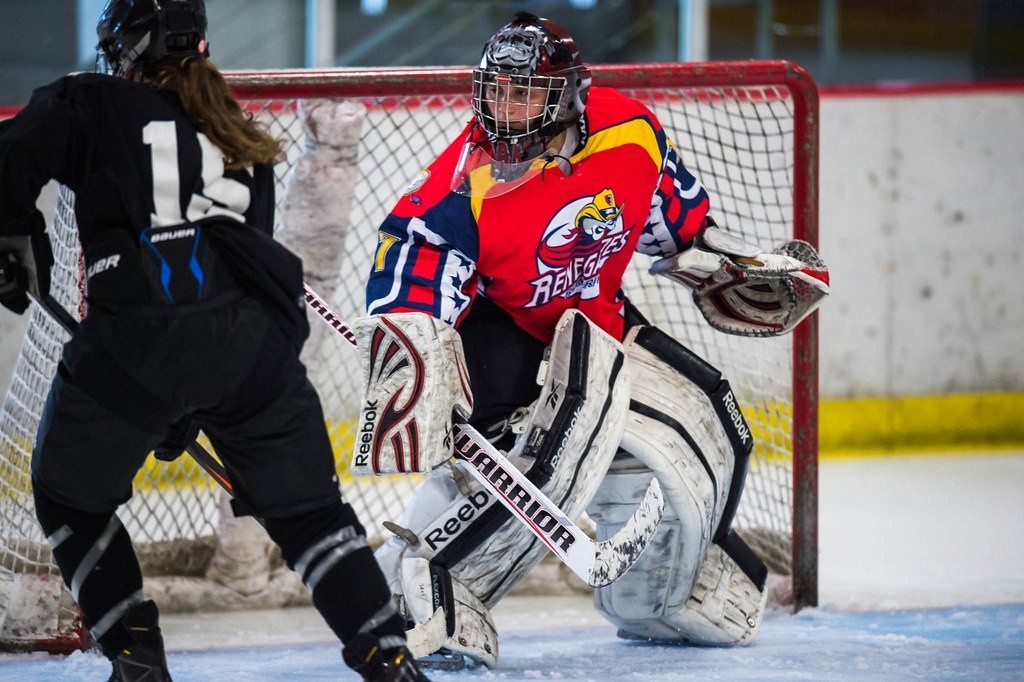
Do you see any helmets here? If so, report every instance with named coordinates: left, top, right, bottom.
left=93, top=0, right=209, bottom=81
left=471, top=10, right=593, bottom=182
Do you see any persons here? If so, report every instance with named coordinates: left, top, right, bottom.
left=0, top=0, right=433, bottom=682
left=350, top=11, right=830, bottom=670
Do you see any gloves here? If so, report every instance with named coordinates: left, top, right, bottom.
left=352, top=311, right=472, bottom=475
left=0, top=208, right=54, bottom=314
left=646, top=225, right=831, bottom=336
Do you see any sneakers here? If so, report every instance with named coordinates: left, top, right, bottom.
left=103, top=598, right=172, bottom=682
left=341, top=632, right=429, bottom=682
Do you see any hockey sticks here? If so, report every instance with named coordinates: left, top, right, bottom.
left=298, top=275, right=666, bottom=592
left=0, top=255, right=449, bottom=662
